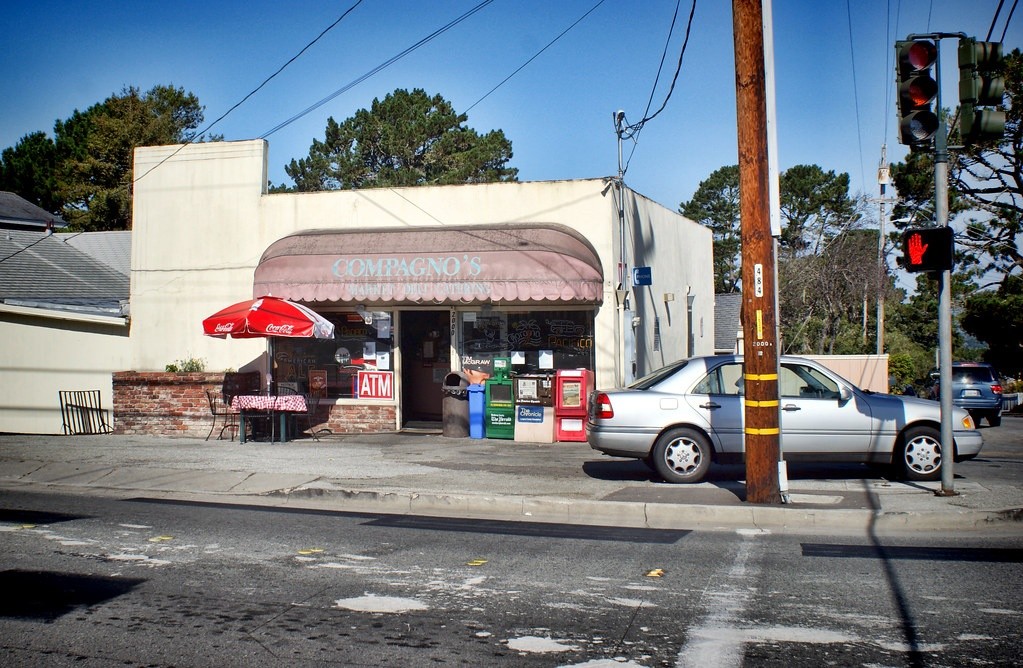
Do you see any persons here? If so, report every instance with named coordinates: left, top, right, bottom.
left=901, top=380, right=941, bottom=401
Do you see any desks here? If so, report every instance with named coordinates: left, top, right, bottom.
left=230, top=393, right=307, bottom=444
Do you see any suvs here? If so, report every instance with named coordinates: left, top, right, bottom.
left=925, top=360, right=1005, bottom=428
left=438, top=339, right=518, bottom=372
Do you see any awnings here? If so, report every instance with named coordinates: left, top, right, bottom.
left=253, top=222, right=604, bottom=302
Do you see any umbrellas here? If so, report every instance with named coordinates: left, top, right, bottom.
left=203, top=297, right=335, bottom=420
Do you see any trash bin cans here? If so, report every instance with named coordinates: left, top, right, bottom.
left=441, top=371, right=470, bottom=438
left=467, top=383, right=485, bottom=439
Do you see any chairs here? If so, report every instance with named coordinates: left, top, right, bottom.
left=205, top=384, right=325, bottom=443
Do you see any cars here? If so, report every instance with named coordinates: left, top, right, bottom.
left=589, top=353, right=985, bottom=484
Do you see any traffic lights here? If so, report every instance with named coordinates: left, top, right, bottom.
left=958, top=38, right=1008, bottom=147
left=897, top=38, right=940, bottom=146
left=898, top=226, right=956, bottom=274
